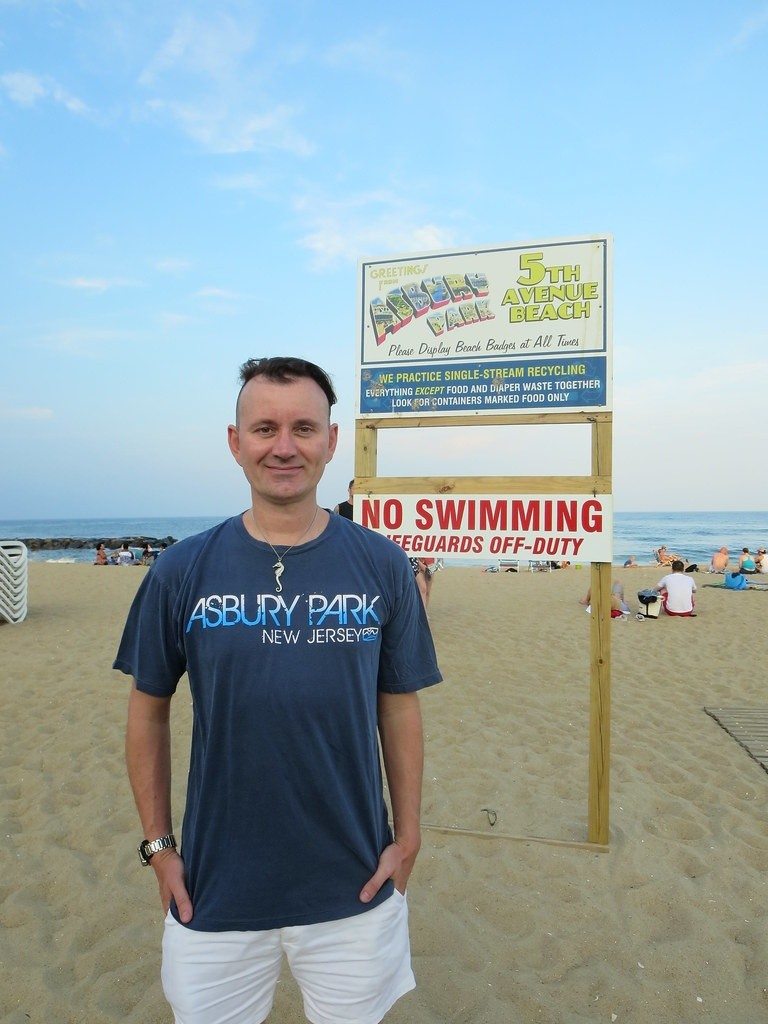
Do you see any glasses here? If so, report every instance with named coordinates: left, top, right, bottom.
left=662, top=547, right=666, bottom=549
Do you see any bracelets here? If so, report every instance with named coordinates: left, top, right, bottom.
left=137, top=834, right=177, bottom=866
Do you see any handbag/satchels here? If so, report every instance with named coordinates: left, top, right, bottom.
left=637, top=589, right=665, bottom=619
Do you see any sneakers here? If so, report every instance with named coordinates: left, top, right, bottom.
left=635, top=613, right=645, bottom=622
left=615, top=614, right=627, bottom=622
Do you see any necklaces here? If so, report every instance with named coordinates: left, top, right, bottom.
left=251, top=507, right=317, bottom=592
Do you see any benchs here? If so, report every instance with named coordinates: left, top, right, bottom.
left=528, top=561, right=551, bottom=573
left=498, top=559, right=520, bottom=572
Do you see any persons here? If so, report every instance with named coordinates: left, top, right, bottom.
left=411, top=558, right=443, bottom=609
left=579, top=580, right=631, bottom=615
left=333, top=480, right=354, bottom=521
left=112, top=358, right=441, bottom=1024
left=709, top=547, right=729, bottom=574
left=547, top=561, right=570, bottom=569
left=654, top=561, right=697, bottom=617
left=738, top=548, right=756, bottom=574
left=754, top=547, right=768, bottom=573
left=658, top=546, right=682, bottom=566
left=624, top=557, right=634, bottom=568
left=96, top=543, right=167, bottom=565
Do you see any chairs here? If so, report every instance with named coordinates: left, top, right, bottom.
left=653, top=550, right=673, bottom=567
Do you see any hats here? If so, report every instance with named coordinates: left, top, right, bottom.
left=757, top=546, right=767, bottom=552
